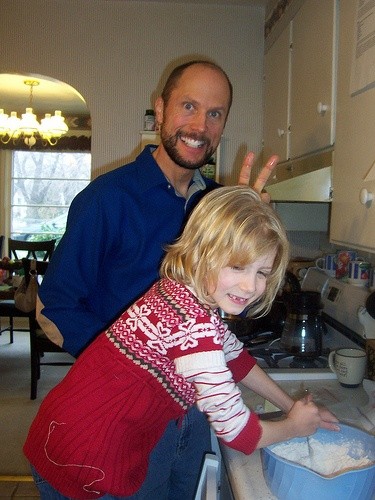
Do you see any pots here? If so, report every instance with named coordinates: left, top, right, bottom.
left=272, top=271, right=301, bottom=313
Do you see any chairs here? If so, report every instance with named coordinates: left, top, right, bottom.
left=0, top=236, right=74, bottom=400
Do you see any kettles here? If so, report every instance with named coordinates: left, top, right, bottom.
left=277, top=289, right=328, bottom=357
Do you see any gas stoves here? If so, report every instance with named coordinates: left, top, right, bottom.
left=221, top=267, right=375, bottom=380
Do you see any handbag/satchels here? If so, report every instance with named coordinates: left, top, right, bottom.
left=15, top=259, right=39, bottom=313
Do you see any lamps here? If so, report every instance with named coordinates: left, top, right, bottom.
left=0, top=80, right=68, bottom=150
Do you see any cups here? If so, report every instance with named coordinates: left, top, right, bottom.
left=315, top=253, right=337, bottom=277
left=336, top=249, right=365, bottom=280
left=328, top=347, right=368, bottom=388
left=347, top=261, right=372, bottom=287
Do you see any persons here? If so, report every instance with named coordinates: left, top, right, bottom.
left=36, top=60, right=280, bottom=500
left=21, top=183, right=341, bottom=500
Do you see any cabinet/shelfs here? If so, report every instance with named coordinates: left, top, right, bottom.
left=139, top=130, right=223, bottom=184
left=265, top=0, right=375, bottom=254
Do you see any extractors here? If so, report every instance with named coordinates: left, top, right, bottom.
left=261, top=149, right=334, bottom=202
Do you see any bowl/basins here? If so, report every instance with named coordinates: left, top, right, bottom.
left=260, top=422, right=375, bottom=500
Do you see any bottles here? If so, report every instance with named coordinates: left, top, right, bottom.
left=144, top=109, right=155, bottom=131
